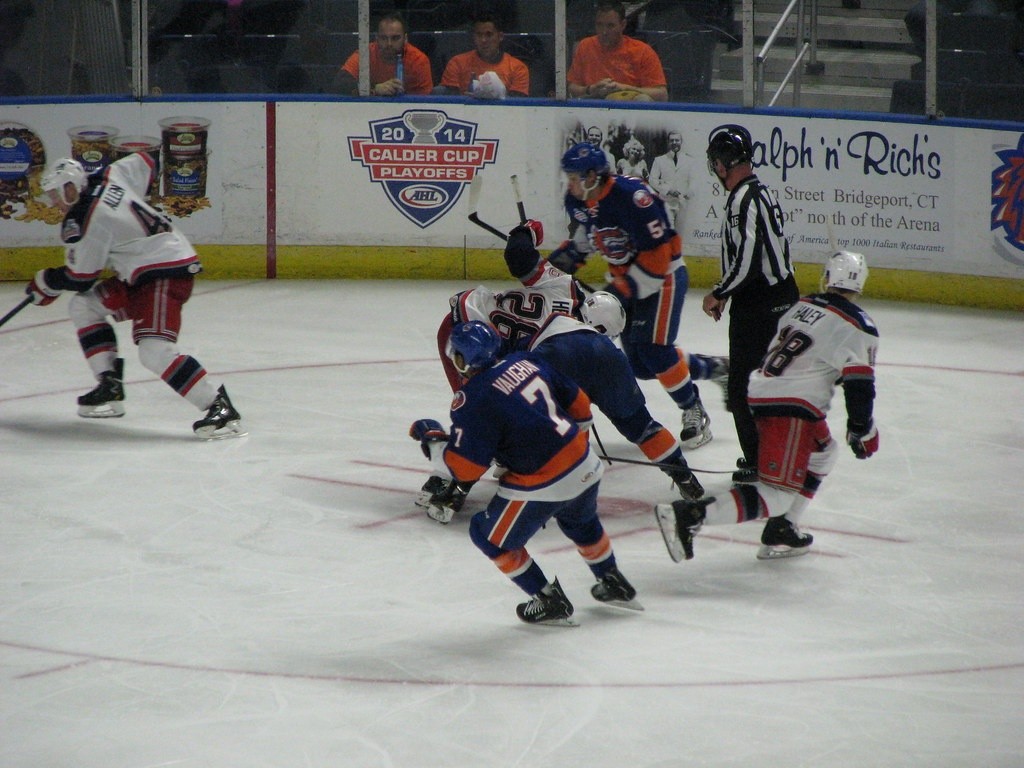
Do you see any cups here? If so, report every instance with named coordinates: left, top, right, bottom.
left=66, top=114, right=212, bottom=199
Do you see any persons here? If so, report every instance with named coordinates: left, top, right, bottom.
left=703, top=124, right=802, bottom=485
left=567, top=0, right=670, bottom=104
left=333, top=12, right=433, bottom=96
left=26, top=152, right=248, bottom=439
left=548, top=142, right=732, bottom=451
left=653, top=250, right=881, bottom=564
left=409, top=220, right=704, bottom=624
left=439, top=13, right=530, bottom=99
left=586, top=126, right=696, bottom=232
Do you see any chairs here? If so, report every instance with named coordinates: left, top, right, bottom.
left=0, top=0, right=740, bottom=102
left=891, top=0, right=1024, bottom=122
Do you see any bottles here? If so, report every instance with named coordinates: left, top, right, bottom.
left=468, top=72, right=476, bottom=93
left=396, top=54, right=404, bottom=86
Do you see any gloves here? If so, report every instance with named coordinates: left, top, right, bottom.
left=25, top=268, right=62, bottom=306
left=519, top=219, right=543, bottom=247
left=547, top=240, right=588, bottom=275
left=846, top=420, right=879, bottom=458
left=409, top=419, right=447, bottom=443
left=603, top=275, right=637, bottom=307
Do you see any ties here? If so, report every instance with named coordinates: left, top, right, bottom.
left=672, top=152, right=678, bottom=167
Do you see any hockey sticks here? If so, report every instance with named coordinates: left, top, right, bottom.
left=597, top=454, right=758, bottom=473
left=510, top=172, right=614, bottom=468
left=465, top=176, right=511, bottom=239
left=0, top=296, right=34, bottom=328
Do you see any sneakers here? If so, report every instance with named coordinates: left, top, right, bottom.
left=655, top=496, right=716, bottom=563
left=427, top=479, right=478, bottom=523
left=736, top=458, right=750, bottom=469
left=591, top=566, right=644, bottom=612
left=757, top=514, right=813, bottom=559
left=77, top=358, right=126, bottom=418
left=415, top=476, right=449, bottom=507
left=669, top=467, right=706, bottom=502
left=695, top=354, right=732, bottom=411
left=516, top=575, right=580, bottom=627
left=493, top=459, right=509, bottom=478
left=680, top=384, right=713, bottom=450
left=193, top=384, right=248, bottom=442
left=731, top=470, right=759, bottom=488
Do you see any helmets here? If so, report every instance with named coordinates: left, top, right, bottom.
left=706, top=124, right=754, bottom=169
left=560, top=142, right=606, bottom=175
left=447, top=320, right=501, bottom=373
left=579, top=290, right=626, bottom=337
left=822, top=251, right=869, bottom=292
left=38, top=158, right=89, bottom=206
